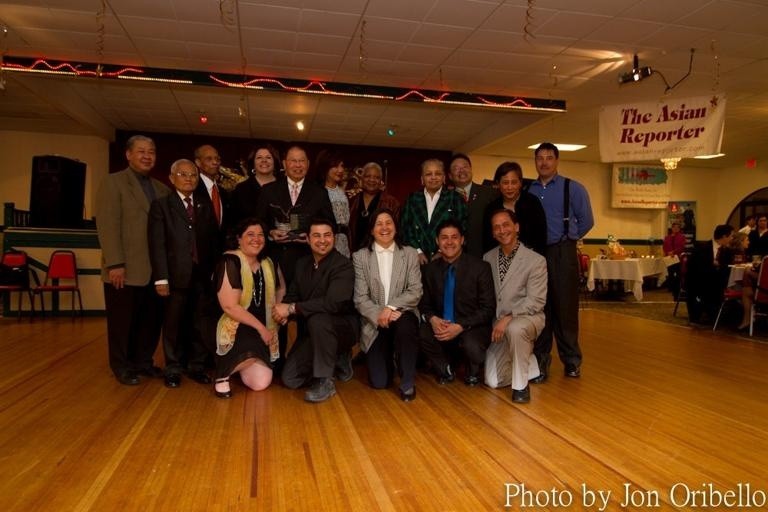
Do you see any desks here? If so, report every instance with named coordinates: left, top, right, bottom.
left=586, top=256, right=668, bottom=301
left=713, top=262, right=754, bottom=332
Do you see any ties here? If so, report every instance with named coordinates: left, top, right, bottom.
left=291, top=183, right=299, bottom=206
left=444, top=266, right=456, bottom=323
left=184, top=196, right=195, bottom=225
left=212, top=183, right=221, bottom=224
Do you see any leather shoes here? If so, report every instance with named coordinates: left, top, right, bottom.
left=214, top=379, right=232, bottom=398
left=112, top=366, right=140, bottom=386
left=143, top=367, right=164, bottom=378
left=440, top=372, right=455, bottom=384
left=165, top=374, right=180, bottom=388
left=401, top=385, right=416, bottom=400
left=186, top=369, right=213, bottom=384
left=464, top=375, right=482, bottom=387
left=564, top=368, right=582, bottom=378
left=529, top=353, right=552, bottom=384
left=512, top=384, right=530, bottom=403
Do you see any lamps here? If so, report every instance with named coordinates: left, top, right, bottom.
left=618, top=52, right=653, bottom=85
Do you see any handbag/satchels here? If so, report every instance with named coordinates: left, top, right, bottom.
left=1, top=266, right=27, bottom=285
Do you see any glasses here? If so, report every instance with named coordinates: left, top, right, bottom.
left=174, top=171, right=198, bottom=178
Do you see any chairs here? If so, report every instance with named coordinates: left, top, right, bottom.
left=30, top=250, right=83, bottom=323
left=749, top=256, right=768, bottom=337
left=673, top=252, right=688, bottom=316
left=0, top=250, right=39, bottom=323
left=578, top=254, right=599, bottom=296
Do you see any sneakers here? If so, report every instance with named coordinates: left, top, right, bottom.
left=337, top=361, right=354, bottom=382
left=304, top=378, right=336, bottom=402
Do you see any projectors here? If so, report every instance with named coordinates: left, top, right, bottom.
left=618, top=66, right=652, bottom=86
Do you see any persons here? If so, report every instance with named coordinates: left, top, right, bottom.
left=683, top=204, right=694, bottom=233
left=95, top=135, right=174, bottom=385
left=481, top=161, right=548, bottom=363
left=522, top=143, right=594, bottom=383
left=663, top=222, right=686, bottom=296
left=147, top=159, right=218, bottom=388
left=483, top=209, right=550, bottom=403
left=211, top=144, right=496, bottom=403
left=685, top=215, right=768, bottom=332
left=194, top=145, right=231, bottom=369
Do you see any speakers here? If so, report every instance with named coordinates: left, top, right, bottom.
left=29, top=155, right=87, bottom=229
